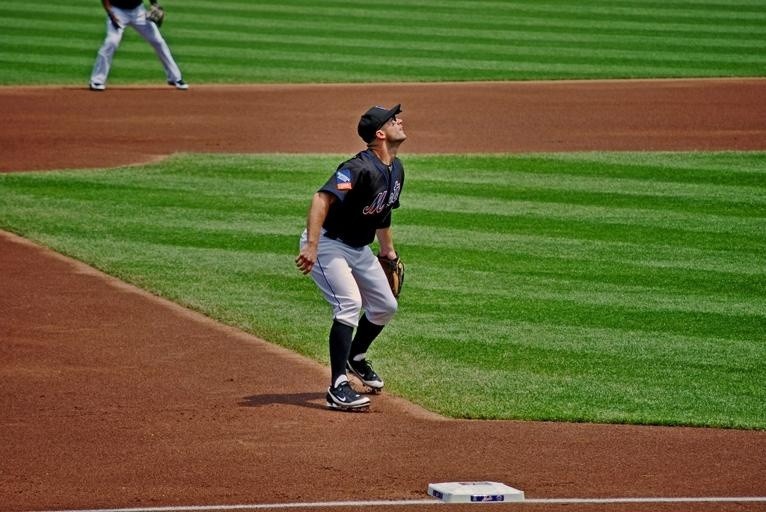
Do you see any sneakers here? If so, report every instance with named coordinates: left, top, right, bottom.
left=88, top=79, right=106, bottom=90
left=325, top=381, right=371, bottom=410
left=346, top=355, right=385, bottom=390
left=166, top=78, right=188, bottom=90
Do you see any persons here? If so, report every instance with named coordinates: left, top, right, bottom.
left=293, top=103, right=412, bottom=408
left=89, top=0, right=189, bottom=91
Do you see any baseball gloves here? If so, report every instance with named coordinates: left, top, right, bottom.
left=146, top=4, right=164, bottom=27
left=377, top=251, right=404, bottom=300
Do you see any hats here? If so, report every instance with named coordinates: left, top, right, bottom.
left=358, top=103, right=402, bottom=139
